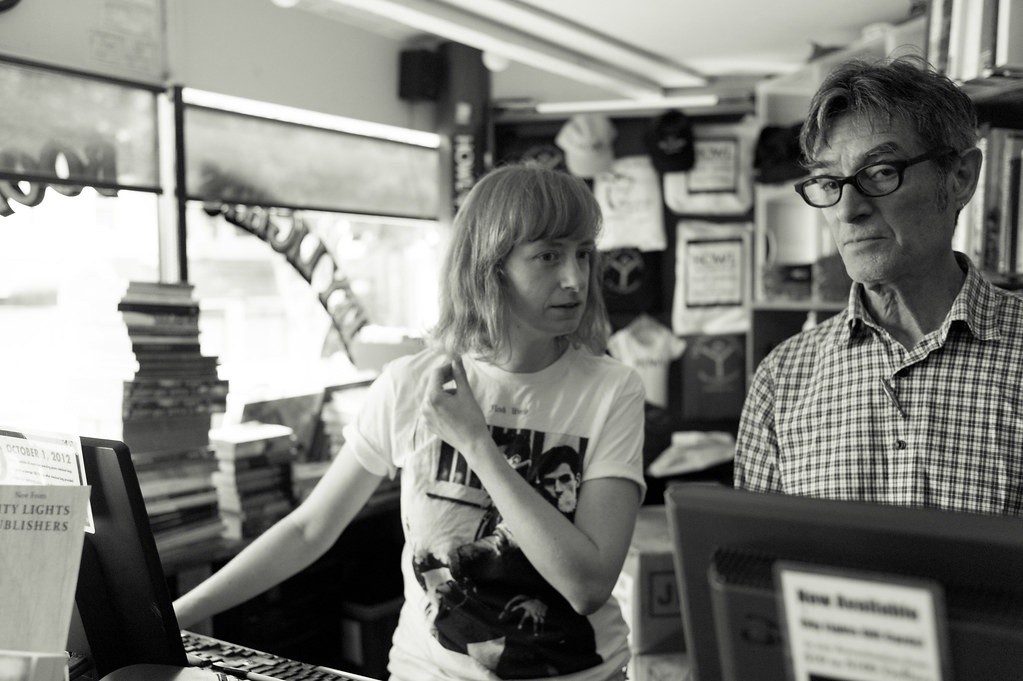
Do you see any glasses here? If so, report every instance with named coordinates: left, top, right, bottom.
left=793, top=149, right=944, bottom=208
left=542, top=474, right=574, bottom=488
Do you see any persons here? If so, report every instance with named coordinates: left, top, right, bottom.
left=731, top=42, right=1023, bottom=516
left=168, top=160, right=652, bottom=681
left=448, top=445, right=604, bottom=680
left=435, top=430, right=543, bottom=654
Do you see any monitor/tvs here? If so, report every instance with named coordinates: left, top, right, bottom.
left=666, top=482, right=1023, bottom=681
left=0, top=427, right=189, bottom=681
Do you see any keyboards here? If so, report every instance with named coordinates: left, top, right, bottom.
left=177, top=629, right=380, bottom=681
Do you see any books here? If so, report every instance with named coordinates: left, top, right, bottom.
left=751, top=0, right=1023, bottom=282
left=82, top=270, right=303, bottom=557
